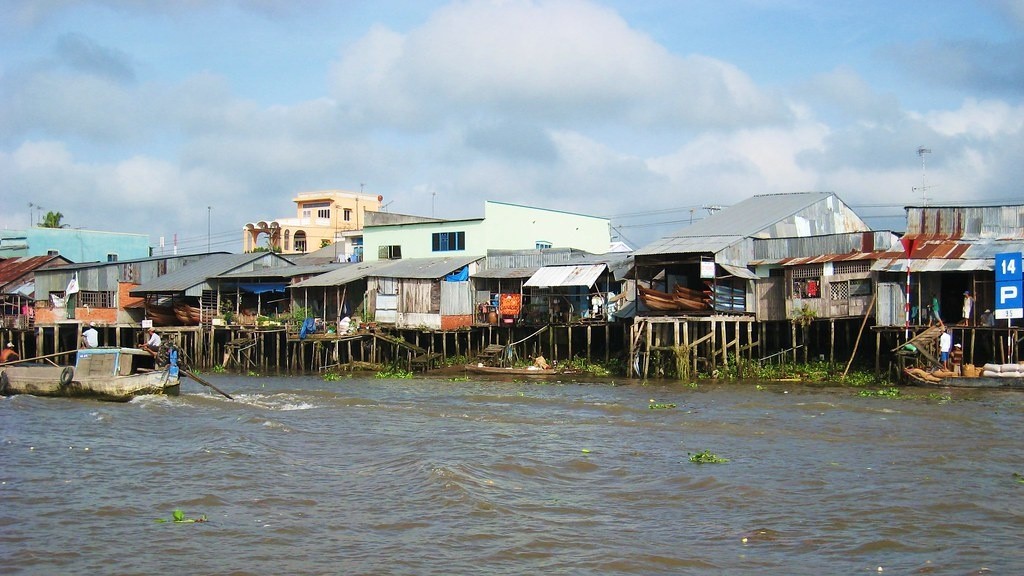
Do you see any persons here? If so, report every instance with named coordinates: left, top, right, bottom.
left=939, top=329, right=950, bottom=370
left=927, top=291, right=944, bottom=326
left=962, top=291, right=974, bottom=326
left=0, top=342, right=20, bottom=363
left=138, top=327, right=161, bottom=352
left=80, top=322, right=98, bottom=348
left=952, top=343, right=962, bottom=376
left=980, top=309, right=994, bottom=327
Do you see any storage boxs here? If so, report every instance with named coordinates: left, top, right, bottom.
left=963, top=365, right=983, bottom=376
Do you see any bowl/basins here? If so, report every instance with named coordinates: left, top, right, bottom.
left=503, top=318, right=514, bottom=323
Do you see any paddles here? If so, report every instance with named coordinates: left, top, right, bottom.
left=144, top=348, right=234, bottom=400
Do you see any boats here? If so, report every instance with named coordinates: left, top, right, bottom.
left=0, top=339, right=183, bottom=403
left=123, top=300, right=217, bottom=325
left=904, top=367, right=1024, bottom=390
left=464, top=363, right=558, bottom=375
left=637, top=280, right=768, bottom=315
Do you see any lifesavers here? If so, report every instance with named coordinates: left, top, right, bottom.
left=60, top=366, right=74, bottom=385
left=0, top=371, right=8, bottom=391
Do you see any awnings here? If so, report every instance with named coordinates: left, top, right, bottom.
left=523, top=263, right=607, bottom=304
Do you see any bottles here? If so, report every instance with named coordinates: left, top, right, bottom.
left=488, top=311, right=497, bottom=324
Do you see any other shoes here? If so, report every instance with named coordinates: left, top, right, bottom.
left=925, top=323, right=931, bottom=327
left=938, top=325, right=945, bottom=331
left=941, top=369, right=949, bottom=372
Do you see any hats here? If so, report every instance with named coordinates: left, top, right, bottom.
left=984, top=309, right=990, bottom=313
left=954, top=343, right=961, bottom=348
left=963, top=291, right=969, bottom=295
left=6, top=341, right=15, bottom=348
left=148, top=327, right=156, bottom=331
left=89, top=321, right=96, bottom=327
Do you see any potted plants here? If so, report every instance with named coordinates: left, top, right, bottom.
left=255, top=316, right=283, bottom=330
left=355, top=307, right=367, bottom=329
left=212, top=300, right=239, bottom=325
left=368, top=313, right=376, bottom=329
